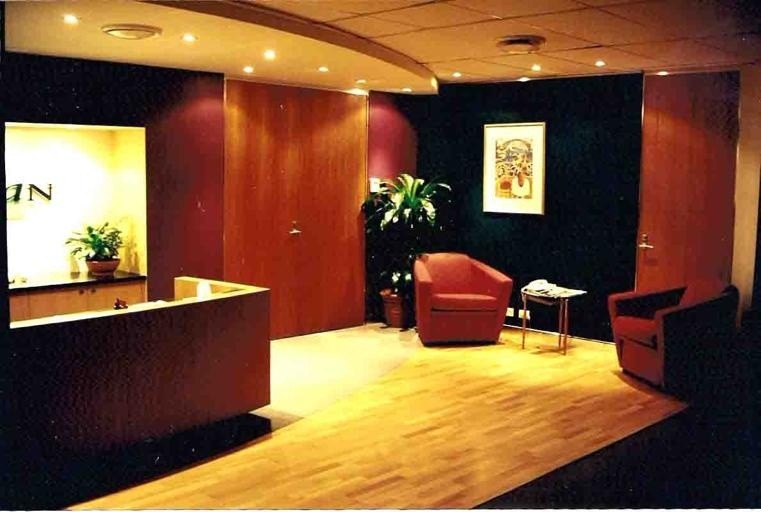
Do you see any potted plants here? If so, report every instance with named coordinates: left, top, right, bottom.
left=359, top=172, right=452, bottom=327
left=65, top=221, right=124, bottom=280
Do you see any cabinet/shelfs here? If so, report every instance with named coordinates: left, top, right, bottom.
left=9, top=279, right=146, bottom=322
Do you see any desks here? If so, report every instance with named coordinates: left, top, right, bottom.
left=521, top=291, right=573, bottom=355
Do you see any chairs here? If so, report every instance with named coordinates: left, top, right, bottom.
left=608, top=275, right=739, bottom=394
left=414, top=252, right=514, bottom=346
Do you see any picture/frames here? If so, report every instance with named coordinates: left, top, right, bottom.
left=482, top=121, right=546, bottom=217
